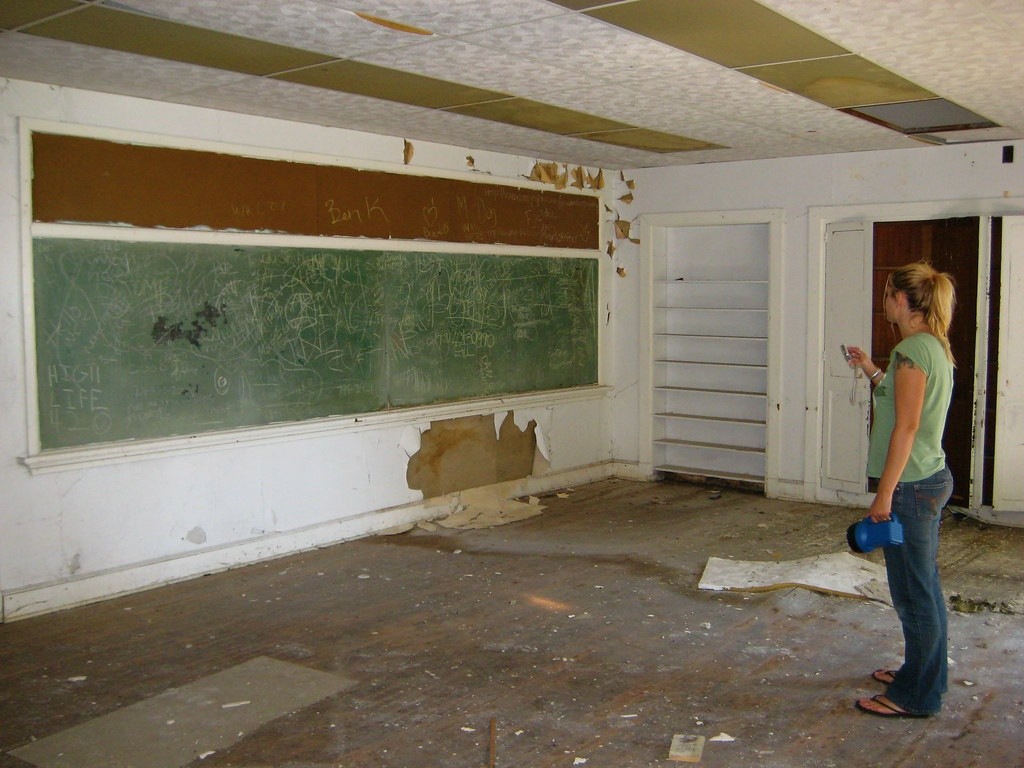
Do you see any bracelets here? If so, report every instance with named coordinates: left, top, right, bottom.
left=871, top=368, right=881, bottom=378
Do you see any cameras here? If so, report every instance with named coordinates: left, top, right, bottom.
left=840, top=345, right=852, bottom=361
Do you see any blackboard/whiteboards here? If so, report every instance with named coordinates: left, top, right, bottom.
left=20, top=224, right=616, bottom=473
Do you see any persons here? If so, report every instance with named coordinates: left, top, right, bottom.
left=845, top=263, right=956, bottom=717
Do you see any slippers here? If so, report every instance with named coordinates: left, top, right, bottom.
left=856, top=695, right=933, bottom=719
left=873, top=668, right=896, bottom=686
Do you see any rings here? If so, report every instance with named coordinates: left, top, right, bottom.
left=883, top=518, right=886, bottom=521
